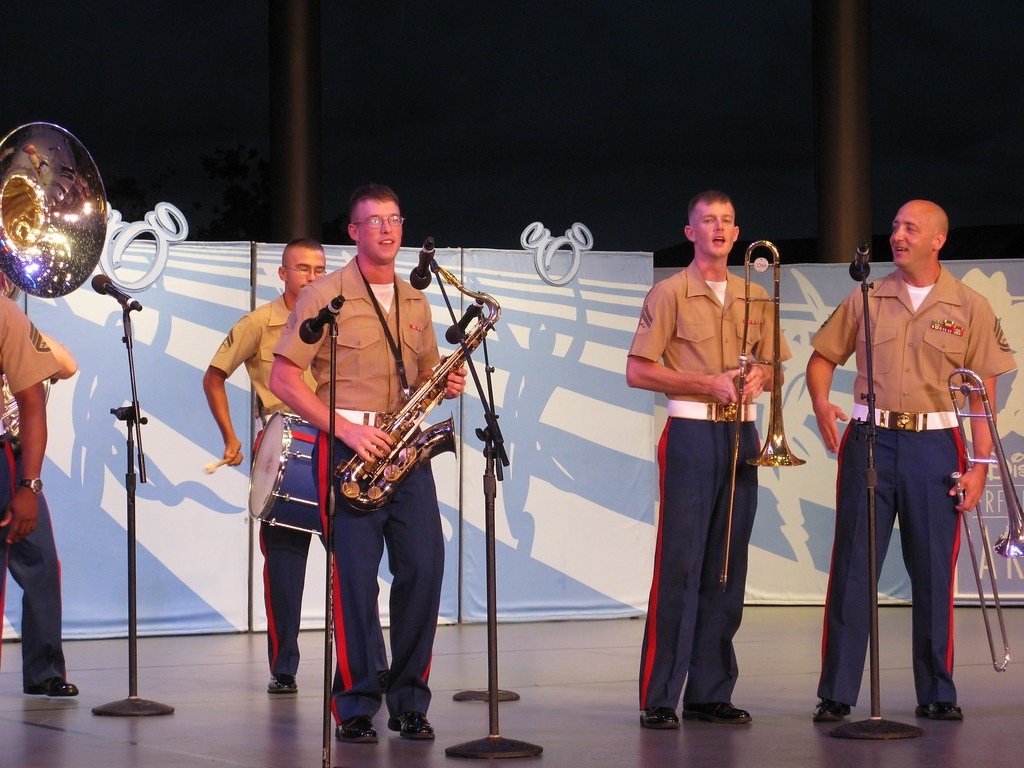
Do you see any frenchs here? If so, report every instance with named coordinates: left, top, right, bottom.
left=1, top=120, right=109, bottom=448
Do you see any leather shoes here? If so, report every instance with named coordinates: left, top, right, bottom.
left=640, top=704, right=680, bottom=728
left=811, top=698, right=844, bottom=720
left=335, top=716, right=379, bottom=743
left=914, top=700, right=963, bottom=720
left=388, top=710, right=435, bottom=739
left=266, top=674, right=298, bottom=694
left=24, top=674, right=78, bottom=696
left=683, top=698, right=752, bottom=724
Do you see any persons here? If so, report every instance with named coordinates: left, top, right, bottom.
left=204, top=239, right=394, bottom=695
left=269, top=184, right=468, bottom=742
left=0, top=294, right=81, bottom=696
left=626, top=190, right=792, bottom=727
left=807, top=198, right=1019, bottom=725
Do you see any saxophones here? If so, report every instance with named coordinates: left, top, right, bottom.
left=332, top=253, right=502, bottom=516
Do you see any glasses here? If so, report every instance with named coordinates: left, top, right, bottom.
left=285, top=264, right=328, bottom=281
left=353, top=215, right=405, bottom=229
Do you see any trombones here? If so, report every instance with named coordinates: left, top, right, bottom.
left=945, top=366, right=1023, bottom=672
left=718, top=240, right=809, bottom=594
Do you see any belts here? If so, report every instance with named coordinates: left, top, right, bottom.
left=336, top=407, right=381, bottom=428
left=851, top=401, right=963, bottom=433
left=668, top=400, right=755, bottom=422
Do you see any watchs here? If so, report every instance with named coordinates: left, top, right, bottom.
left=18, top=477, right=43, bottom=493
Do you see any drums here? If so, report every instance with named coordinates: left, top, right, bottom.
left=246, top=408, right=323, bottom=535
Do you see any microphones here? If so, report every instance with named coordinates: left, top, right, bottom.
left=445, top=293, right=485, bottom=345
left=299, top=295, right=346, bottom=345
left=410, top=237, right=435, bottom=290
left=848, top=241, right=870, bottom=281
left=91, top=274, right=143, bottom=312
